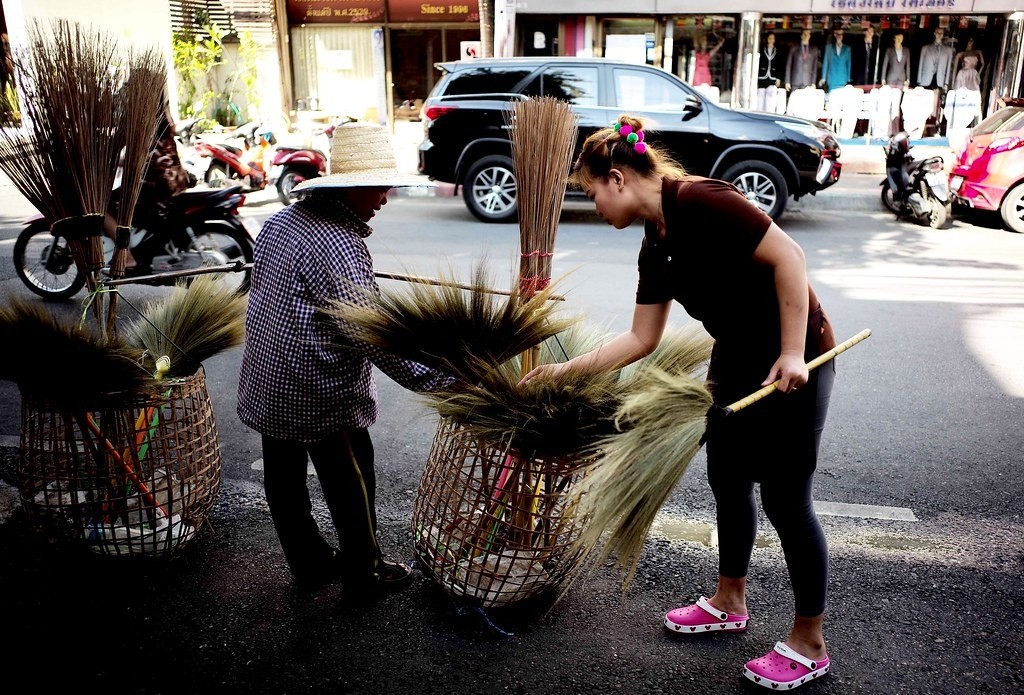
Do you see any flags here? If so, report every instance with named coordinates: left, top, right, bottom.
left=761, top=12, right=980, bottom=29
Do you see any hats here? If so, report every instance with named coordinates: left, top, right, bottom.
left=288, top=121, right=439, bottom=192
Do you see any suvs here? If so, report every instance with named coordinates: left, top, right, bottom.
left=414, top=59, right=842, bottom=225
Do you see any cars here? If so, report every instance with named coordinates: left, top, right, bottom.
left=946, top=97, right=1024, bottom=234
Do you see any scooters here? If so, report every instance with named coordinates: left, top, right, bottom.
left=881, top=127, right=951, bottom=229
left=191, top=116, right=277, bottom=193
left=265, top=114, right=360, bottom=207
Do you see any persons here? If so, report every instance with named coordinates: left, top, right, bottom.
left=236, top=122, right=439, bottom=593
left=687, top=27, right=724, bottom=106
left=515, top=115, right=836, bottom=691
left=757, top=27, right=984, bottom=142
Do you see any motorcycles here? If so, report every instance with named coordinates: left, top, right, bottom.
left=14, top=147, right=255, bottom=304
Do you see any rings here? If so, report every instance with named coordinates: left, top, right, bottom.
left=793, top=386, right=798, bottom=391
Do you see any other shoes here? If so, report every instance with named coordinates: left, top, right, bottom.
left=293, top=547, right=340, bottom=580
left=341, top=558, right=413, bottom=584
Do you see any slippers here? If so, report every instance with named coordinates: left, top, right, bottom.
left=663, top=596, right=750, bottom=634
left=742, top=641, right=831, bottom=691
